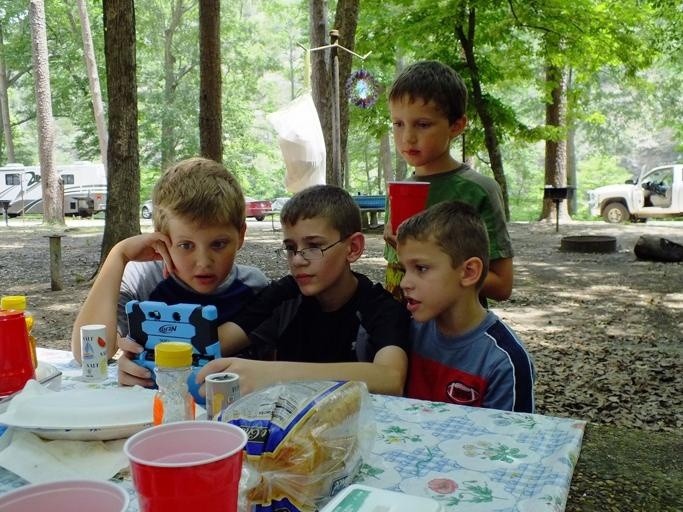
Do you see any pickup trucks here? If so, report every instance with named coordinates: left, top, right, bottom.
left=584, top=163, right=682, bottom=224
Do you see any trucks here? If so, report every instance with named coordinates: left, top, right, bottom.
left=0, top=162, right=107, bottom=218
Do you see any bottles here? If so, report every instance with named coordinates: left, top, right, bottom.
left=152, top=341, right=196, bottom=426
left=0, top=295, right=38, bottom=371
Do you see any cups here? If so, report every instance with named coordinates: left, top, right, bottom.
left=0, top=478, right=130, bottom=512
left=122, top=417, right=248, bottom=512
left=0, top=309, right=38, bottom=397
left=388, top=181, right=430, bottom=236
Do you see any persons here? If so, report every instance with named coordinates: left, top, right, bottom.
left=117, top=185, right=410, bottom=400
left=394, top=202, right=537, bottom=414
left=72, top=158, right=271, bottom=362
left=384, top=61, right=513, bottom=311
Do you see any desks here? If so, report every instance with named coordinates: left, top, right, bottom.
left=0, top=345, right=683, bottom=512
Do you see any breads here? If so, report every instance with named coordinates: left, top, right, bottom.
left=258, top=383, right=362, bottom=510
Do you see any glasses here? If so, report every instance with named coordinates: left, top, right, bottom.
left=274, top=232, right=351, bottom=261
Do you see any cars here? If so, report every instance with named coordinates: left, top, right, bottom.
left=141, top=199, right=153, bottom=219
left=243, top=196, right=272, bottom=221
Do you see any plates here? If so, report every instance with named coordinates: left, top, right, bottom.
left=1, top=422, right=154, bottom=441
